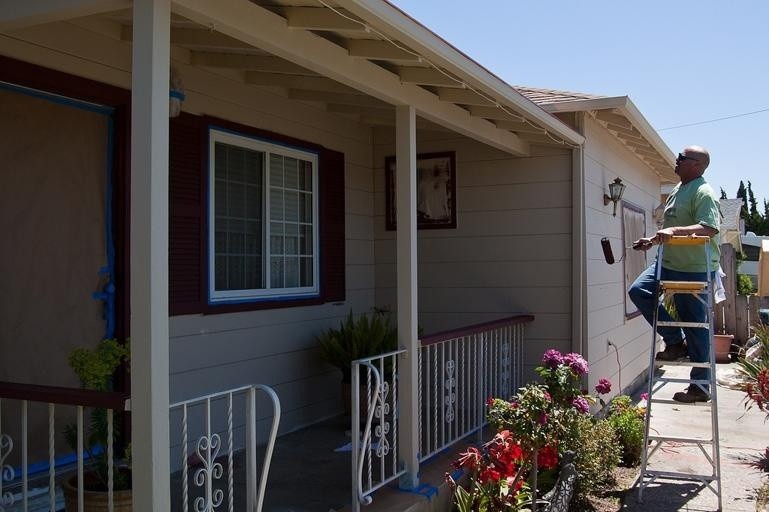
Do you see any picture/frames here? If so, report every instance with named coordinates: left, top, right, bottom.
left=385, top=150, right=457, bottom=229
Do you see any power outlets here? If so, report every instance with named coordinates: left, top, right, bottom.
left=607, top=335, right=613, bottom=351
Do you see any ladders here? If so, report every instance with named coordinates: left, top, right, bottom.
left=638, top=235, right=723, bottom=512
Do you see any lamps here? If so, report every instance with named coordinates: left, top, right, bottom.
left=603, top=176, right=626, bottom=217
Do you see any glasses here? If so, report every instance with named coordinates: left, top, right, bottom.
left=678, top=153, right=700, bottom=161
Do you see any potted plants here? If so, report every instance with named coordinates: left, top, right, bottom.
left=60, top=338, right=132, bottom=512
left=314, top=306, right=424, bottom=424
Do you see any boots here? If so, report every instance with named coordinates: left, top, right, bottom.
left=655, top=336, right=689, bottom=361
left=672, top=384, right=710, bottom=403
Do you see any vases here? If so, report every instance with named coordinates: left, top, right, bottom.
left=710, top=335, right=734, bottom=363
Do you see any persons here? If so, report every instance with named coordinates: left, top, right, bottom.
left=627, top=146, right=721, bottom=404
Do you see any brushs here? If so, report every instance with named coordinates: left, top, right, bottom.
left=601, top=235, right=656, bottom=264
